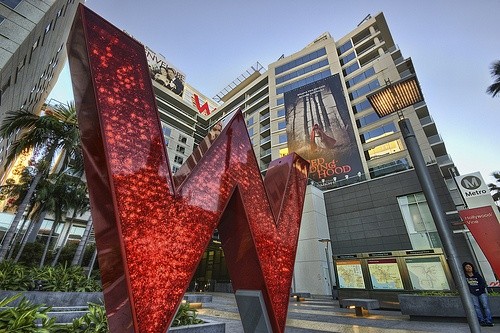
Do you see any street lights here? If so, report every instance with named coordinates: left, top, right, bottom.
left=365, top=74, right=485, bottom=333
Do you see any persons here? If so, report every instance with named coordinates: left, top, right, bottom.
left=312, top=124, right=327, bottom=153
left=153, top=65, right=184, bottom=96
left=462, top=261, right=496, bottom=327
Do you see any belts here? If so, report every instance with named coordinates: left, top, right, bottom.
left=315, top=135, right=321, bottom=137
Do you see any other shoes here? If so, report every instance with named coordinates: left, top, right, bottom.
left=481, top=322, right=492, bottom=326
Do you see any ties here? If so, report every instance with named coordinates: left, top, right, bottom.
left=169, top=79, right=173, bottom=83
